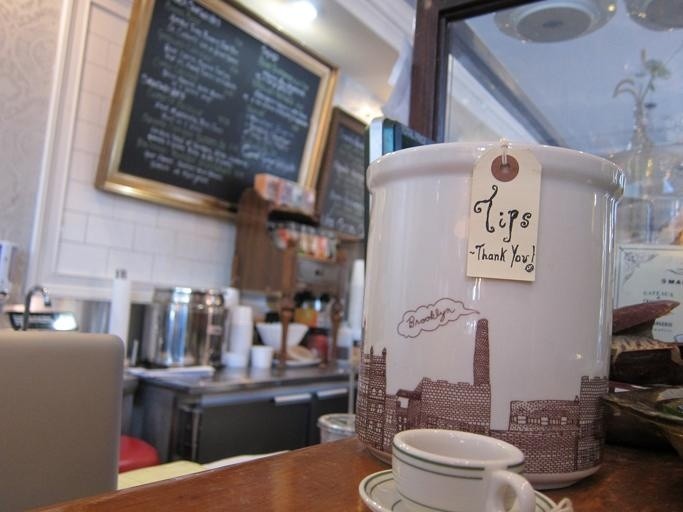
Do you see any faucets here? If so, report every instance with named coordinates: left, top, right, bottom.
left=20, top=285, right=52, bottom=332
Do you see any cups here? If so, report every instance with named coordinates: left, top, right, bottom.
left=315, top=409, right=357, bottom=447
left=250, top=345, right=274, bottom=369
left=222, top=286, right=252, bottom=368
left=390, top=427, right=536, bottom=512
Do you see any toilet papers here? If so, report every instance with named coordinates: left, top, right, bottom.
left=108, top=277, right=132, bottom=367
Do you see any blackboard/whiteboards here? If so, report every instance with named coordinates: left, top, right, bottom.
left=95, top=0, right=370, bottom=241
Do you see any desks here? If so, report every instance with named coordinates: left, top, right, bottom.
left=33, top=419, right=682, bottom=512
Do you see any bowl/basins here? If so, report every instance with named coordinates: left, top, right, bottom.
left=255, top=320, right=309, bottom=353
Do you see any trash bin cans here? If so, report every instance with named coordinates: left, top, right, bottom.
left=316, top=413, right=356, bottom=444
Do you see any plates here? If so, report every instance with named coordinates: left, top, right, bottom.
left=598, top=384, right=683, bottom=462
left=271, top=358, right=322, bottom=366
left=356, top=467, right=561, bottom=512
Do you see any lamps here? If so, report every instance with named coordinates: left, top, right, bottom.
left=495, top=0, right=682, bottom=45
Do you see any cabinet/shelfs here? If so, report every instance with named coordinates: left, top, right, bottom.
left=125, top=175, right=359, bottom=462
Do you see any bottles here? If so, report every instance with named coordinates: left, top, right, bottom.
left=334, top=319, right=353, bottom=370
left=307, top=326, right=330, bottom=363
left=267, top=288, right=343, bottom=330
